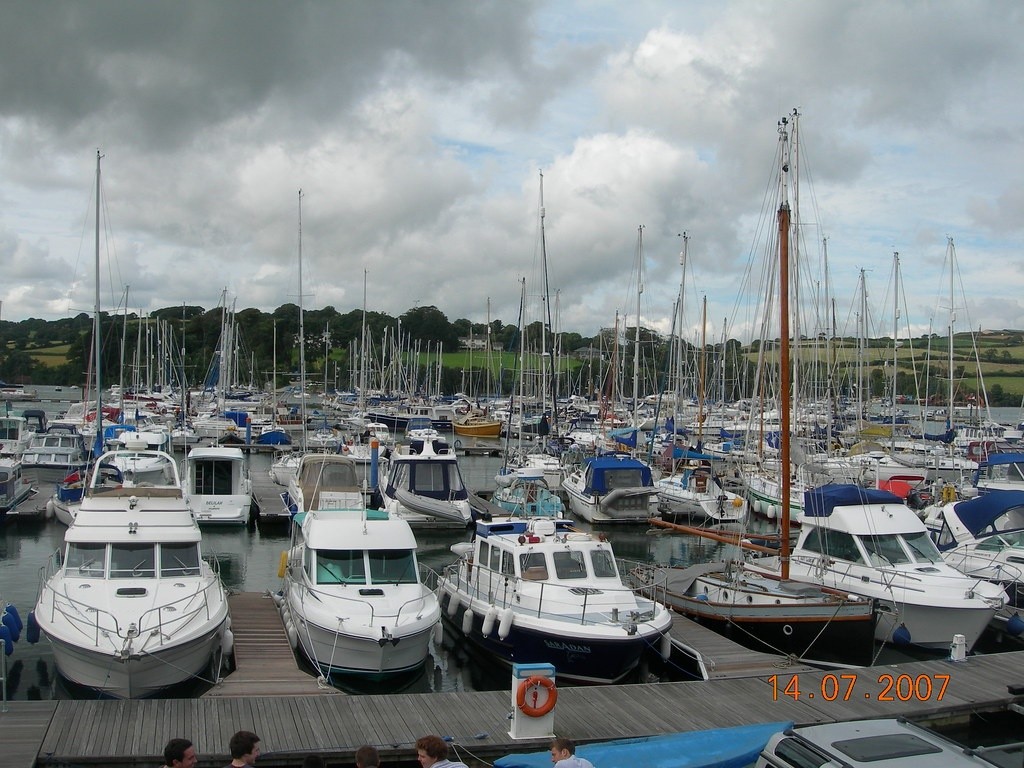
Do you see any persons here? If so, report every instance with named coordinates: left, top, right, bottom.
left=355, top=745, right=380, bottom=768
left=223, top=731, right=261, bottom=768
left=157, top=738, right=197, bottom=768
left=304, top=755, right=324, bottom=768
left=416, top=735, right=469, bottom=768
left=549, top=739, right=593, bottom=768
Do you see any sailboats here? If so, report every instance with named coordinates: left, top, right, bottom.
left=0, top=107, right=1023, bottom=698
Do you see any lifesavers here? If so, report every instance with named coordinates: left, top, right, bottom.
left=563, top=532, right=593, bottom=541
left=516, top=675, right=558, bottom=718
left=519, top=533, right=546, bottom=544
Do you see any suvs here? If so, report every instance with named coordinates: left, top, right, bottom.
left=754, top=716, right=1006, bottom=768
